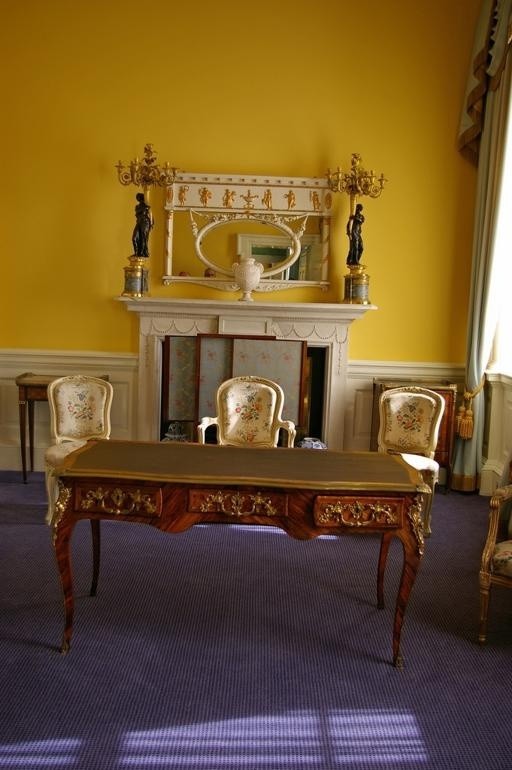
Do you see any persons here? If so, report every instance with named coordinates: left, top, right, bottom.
left=312, top=191, right=321, bottom=211
left=205, top=268, right=216, bottom=278
left=284, top=190, right=296, bottom=210
left=178, top=185, right=189, bottom=206
left=200, top=187, right=209, bottom=208
left=221, top=189, right=235, bottom=209
left=130, top=193, right=154, bottom=258
left=265, top=189, right=272, bottom=209
left=346, top=203, right=365, bottom=265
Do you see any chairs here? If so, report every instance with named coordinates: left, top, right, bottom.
left=377, top=387, right=445, bottom=538
left=45, top=376, right=113, bottom=525
left=198, top=376, right=297, bottom=449
left=477, top=484, right=512, bottom=641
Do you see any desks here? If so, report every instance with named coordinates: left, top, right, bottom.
left=14, top=373, right=108, bottom=484
left=371, top=377, right=458, bottom=495
left=53, top=437, right=432, bottom=671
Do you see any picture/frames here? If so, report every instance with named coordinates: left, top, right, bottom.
left=237, top=232, right=322, bottom=280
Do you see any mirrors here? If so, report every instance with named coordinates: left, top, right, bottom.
left=162, top=170, right=331, bottom=293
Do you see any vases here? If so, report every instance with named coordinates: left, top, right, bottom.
left=296, top=437, right=327, bottom=449
left=232, top=259, right=264, bottom=301
